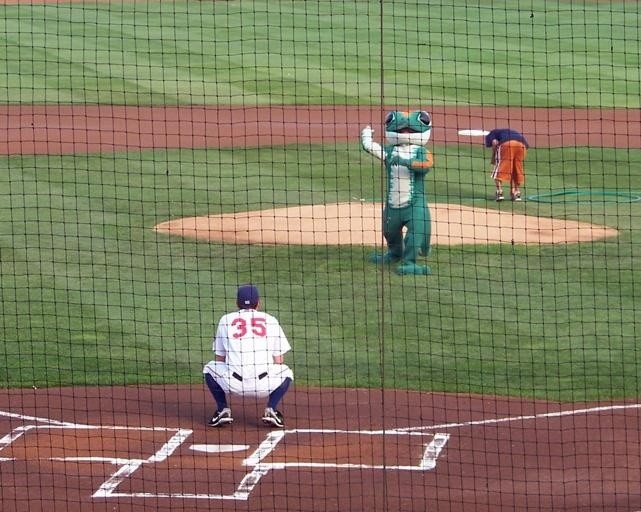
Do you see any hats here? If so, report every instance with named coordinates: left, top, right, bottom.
left=237, top=285, right=259, bottom=308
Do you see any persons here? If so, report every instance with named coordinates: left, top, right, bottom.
left=484, top=129, right=530, bottom=203
left=203, top=284, right=294, bottom=428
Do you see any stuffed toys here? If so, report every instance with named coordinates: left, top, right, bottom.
left=360, top=110, right=435, bottom=277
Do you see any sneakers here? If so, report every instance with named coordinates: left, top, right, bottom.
left=261, top=408, right=285, bottom=427
left=208, top=408, right=233, bottom=427
left=496, top=191, right=522, bottom=201
